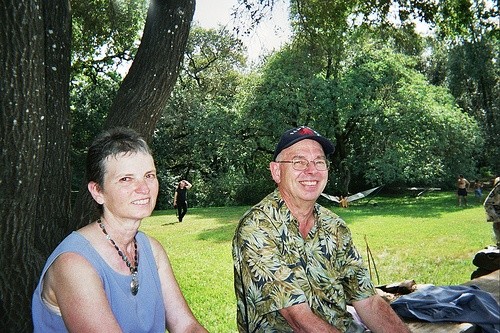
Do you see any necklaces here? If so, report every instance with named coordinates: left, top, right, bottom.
left=97, top=217, right=140, bottom=297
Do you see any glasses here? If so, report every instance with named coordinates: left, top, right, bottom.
left=276, top=159, right=332, bottom=170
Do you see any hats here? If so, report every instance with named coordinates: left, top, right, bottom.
left=274, top=125, right=334, bottom=161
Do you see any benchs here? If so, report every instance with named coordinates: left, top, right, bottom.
left=390, top=270, right=500, bottom=333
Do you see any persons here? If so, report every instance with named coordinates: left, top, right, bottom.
left=484, top=180, right=500, bottom=248
left=231, top=126, right=414, bottom=333
left=473, top=179, right=483, bottom=202
left=30, top=125, right=208, bottom=333
left=174, top=180, right=192, bottom=223
left=493, top=170, right=500, bottom=186
left=457, top=175, right=470, bottom=207
